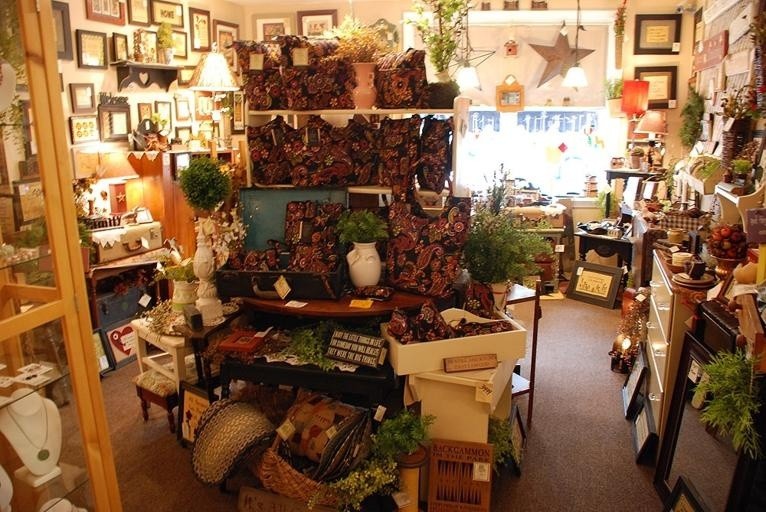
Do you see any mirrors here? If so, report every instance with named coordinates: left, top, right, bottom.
left=653, top=330, right=765, bottom=511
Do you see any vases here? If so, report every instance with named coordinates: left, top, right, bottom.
left=615, top=36, right=623, bottom=69
left=171, top=281, right=198, bottom=314
left=724, top=119, right=748, bottom=171
left=230, top=250, right=243, bottom=267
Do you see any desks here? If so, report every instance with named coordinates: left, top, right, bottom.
left=82, top=247, right=169, bottom=332
left=575, top=220, right=634, bottom=302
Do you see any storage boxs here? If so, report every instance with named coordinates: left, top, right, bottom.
left=380, top=307, right=527, bottom=376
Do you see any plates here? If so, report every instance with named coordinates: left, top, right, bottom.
left=671, top=272, right=714, bottom=289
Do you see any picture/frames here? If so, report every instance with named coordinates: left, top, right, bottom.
left=18, top=98, right=37, bottom=161
left=128, top=0, right=152, bottom=27
left=99, top=152, right=136, bottom=178
left=231, top=91, right=245, bottom=135
left=103, top=316, right=146, bottom=370
left=68, top=115, right=100, bottom=145
left=297, top=9, right=337, bottom=35
left=151, top=0, right=184, bottom=28
left=634, top=14, right=681, bottom=55
left=71, top=146, right=99, bottom=180
left=251, top=11, right=296, bottom=43
left=50, top=0, right=74, bottom=61
left=664, top=476, right=708, bottom=512
left=497, top=81, right=525, bottom=112
left=623, top=343, right=647, bottom=416
left=13, top=177, right=49, bottom=226
left=188, top=7, right=212, bottom=51
left=173, top=152, right=195, bottom=180
left=221, top=330, right=266, bottom=354
left=69, top=83, right=98, bottom=114
left=139, top=103, right=153, bottom=128
left=504, top=404, right=529, bottom=479
left=176, top=98, right=192, bottom=121
left=155, top=100, right=172, bottom=132
left=565, top=260, right=625, bottom=309
left=212, top=18, right=240, bottom=44
left=635, top=66, right=677, bottom=110
left=716, top=273, right=738, bottom=303
left=98, top=90, right=132, bottom=142
left=694, top=7, right=703, bottom=55
left=91, top=327, right=115, bottom=378
left=76, top=28, right=108, bottom=69
left=630, top=400, right=657, bottom=465
left=112, top=32, right=129, bottom=62
left=173, top=30, right=188, bottom=59
left=86, top=0, right=125, bottom=26
left=201, top=121, right=220, bottom=146
left=194, top=92, right=214, bottom=120
left=752, top=131, right=763, bottom=163
left=177, top=381, right=219, bottom=448
left=142, top=28, right=159, bottom=63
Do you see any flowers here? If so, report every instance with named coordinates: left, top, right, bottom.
left=614, top=0, right=630, bottom=35
left=716, top=83, right=755, bottom=118
left=198, top=207, right=255, bottom=246
left=149, top=238, right=196, bottom=284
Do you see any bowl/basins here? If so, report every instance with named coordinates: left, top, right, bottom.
left=665, top=259, right=692, bottom=274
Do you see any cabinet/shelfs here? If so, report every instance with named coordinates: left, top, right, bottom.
left=606, top=164, right=665, bottom=223
left=639, top=246, right=766, bottom=468
left=511, top=229, right=564, bottom=291
left=118, top=61, right=184, bottom=92
left=630, top=209, right=710, bottom=289
left=1, top=0, right=122, bottom=512
left=205, top=279, right=544, bottom=430
left=660, top=300, right=765, bottom=512
left=125, top=140, right=247, bottom=303
left=242, top=97, right=474, bottom=210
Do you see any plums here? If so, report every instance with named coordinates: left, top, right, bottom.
left=708, top=223, right=747, bottom=259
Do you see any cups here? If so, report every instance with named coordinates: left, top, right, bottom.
left=685, top=260, right=706, bottom=280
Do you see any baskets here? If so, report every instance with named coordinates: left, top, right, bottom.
left=234, top=385, right=397, bottom=506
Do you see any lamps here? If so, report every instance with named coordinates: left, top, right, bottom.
left=457, top=61, right=480, bottom=92
left=82, top=150, right=140, bottom=220
left=633, top=110, right=668, bottom=141
left=621, top=80, right=650, bottom=150
left=562, top=63, right=588, bottom=88
left=189, top=43, right=238, bottom=149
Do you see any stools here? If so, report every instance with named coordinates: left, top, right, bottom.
left=132, top=317, right=195, bottom=385
left=133, top=353, right=221, bottom=433
left=410, top=358, right=512, bottom=443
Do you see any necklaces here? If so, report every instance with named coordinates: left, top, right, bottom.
left=6, top=395, right=50, bottom=461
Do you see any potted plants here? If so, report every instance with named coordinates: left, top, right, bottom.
left=462, top=210, right=553, bottom=311
left=630, top=148, right=643, bottom=170
left=407, top=1, right=478, bottom=96
left=338, top=209, right=390, bottom=290
left=321, top=19, right=397, bottom=109
left=158, top=23, right=176, bottom=64
left=732, top=161, right=751, bottom=185
